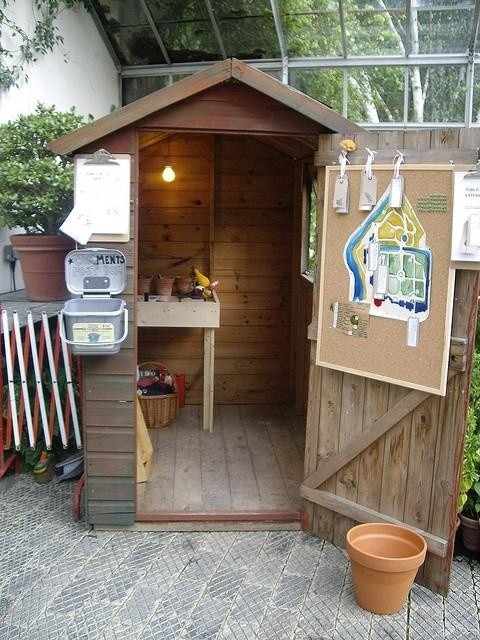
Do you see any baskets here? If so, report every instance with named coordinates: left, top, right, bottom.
left=138, top=362, right=177, bottom=428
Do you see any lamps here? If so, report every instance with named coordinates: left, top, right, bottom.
left=162, top=139, right=176, bottom=182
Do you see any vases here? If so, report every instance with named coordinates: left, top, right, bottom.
left=138, top=274, right=195, bottom=296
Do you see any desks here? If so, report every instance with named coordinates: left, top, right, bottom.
left=137, top=290, right=221, bottom=433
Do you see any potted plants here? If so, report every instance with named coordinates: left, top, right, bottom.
left=0, top=100, right=95, bottom=302
left=460, top=351, right=480, bottom=554
